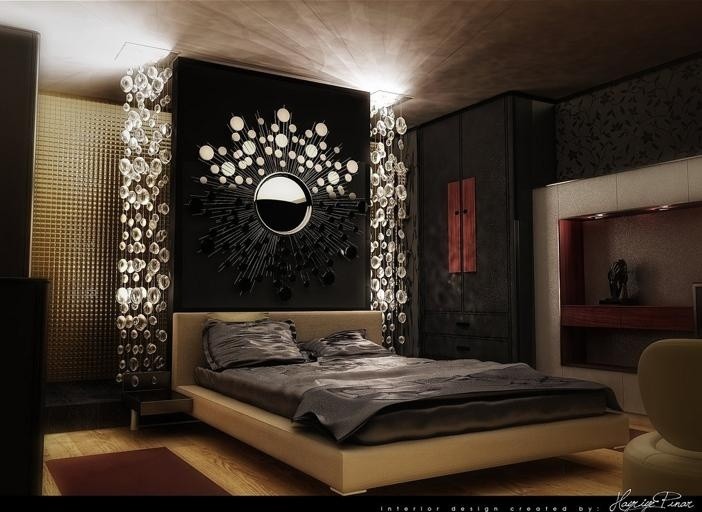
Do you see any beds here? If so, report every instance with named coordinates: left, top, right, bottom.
left=168, top=307, right=631, bottom=501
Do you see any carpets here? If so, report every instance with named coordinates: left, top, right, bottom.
left=45, top=446, right=234, bottom=495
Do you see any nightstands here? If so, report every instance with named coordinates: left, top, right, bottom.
left=121, top=371, right=194, bottom=432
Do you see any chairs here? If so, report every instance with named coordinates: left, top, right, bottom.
left=622, top=337, right=702, bottom=498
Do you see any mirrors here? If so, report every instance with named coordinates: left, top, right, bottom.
left=185, top=103, right=369, bottom=301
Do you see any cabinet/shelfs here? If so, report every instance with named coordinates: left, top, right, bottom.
left=398, top=91, right=558, bottom=362
left=0, top=274, right=55, bottom=498
left=551, top=193, right=701, bottom=375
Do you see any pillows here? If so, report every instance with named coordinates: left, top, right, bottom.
left=202, top=316, right=304, bottom=374
left=298, top=326, right=388, bottom=357
